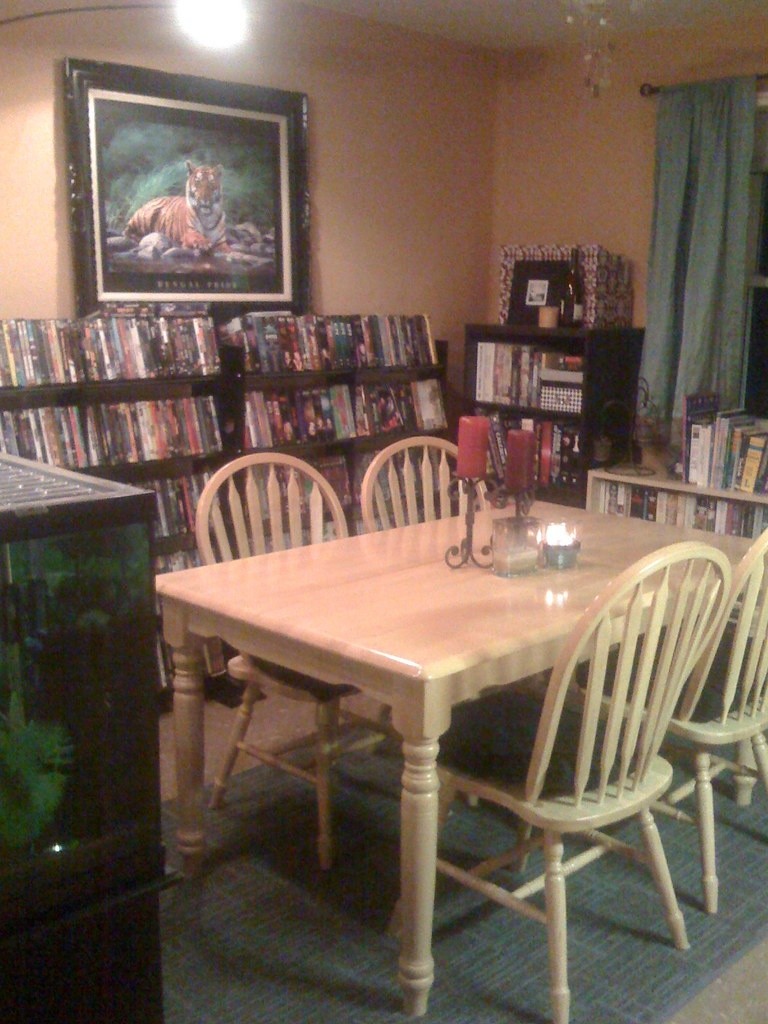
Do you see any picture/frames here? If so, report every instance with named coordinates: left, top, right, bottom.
left=64, top=57, right=311, bottom=316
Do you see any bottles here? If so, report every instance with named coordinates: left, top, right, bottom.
left=560, top=247, right=584, bottom=329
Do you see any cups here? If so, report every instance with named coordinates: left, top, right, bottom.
left=538, top=306, right=560, bottom=329
left=543, top=516, right=576, bottom=571
left=491, top=516, right=542, bottom=578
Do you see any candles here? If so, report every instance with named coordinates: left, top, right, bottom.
left=457, top=416, right=490, bottom=475
left=505, top=430, right=535, bottom=492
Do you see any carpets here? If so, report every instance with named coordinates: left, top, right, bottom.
left=159, top=609, right=768, bottom=1023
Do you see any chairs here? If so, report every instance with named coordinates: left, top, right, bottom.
left=195, top=437, right=768, bottom=1024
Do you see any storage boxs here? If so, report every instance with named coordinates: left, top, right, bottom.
left=498, top=244, right=633, bottom=327
left=0, top=451, right=166, bottom=1024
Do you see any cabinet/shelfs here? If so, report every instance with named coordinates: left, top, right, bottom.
left=465, top=324, right=647, bottom=510
left=0, top=339, right=454, bottom=709
left=586, top=467, right=768, bottom=530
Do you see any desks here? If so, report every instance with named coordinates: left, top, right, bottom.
left=155, top=497, right=768, bottom=1017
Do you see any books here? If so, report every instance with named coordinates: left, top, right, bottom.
left=219, top=311, right=451, bottom=530
left=726, top=591, right=763, bottom=637
left=599, top=480, right=768, bottom=539
left=474, top=406, right=581, bottom=485
left=1, top=314, right=227, bottom=693
left=475, top=342, right=567, bottom=409
left=682, top=390, right=768, bottom=495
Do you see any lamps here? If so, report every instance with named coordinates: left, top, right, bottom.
left=561, top=0, right=646, bottom=99
